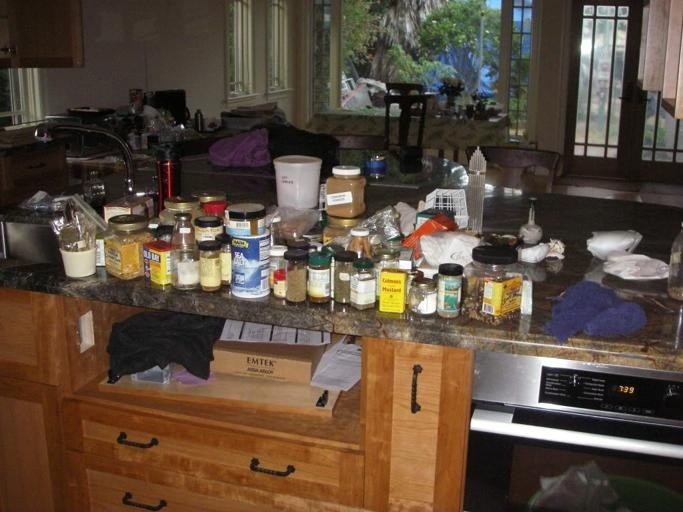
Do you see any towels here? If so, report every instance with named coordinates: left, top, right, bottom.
left=544, top=280, right=647, bottom=342
left=207, top=127, right=271, bottom=169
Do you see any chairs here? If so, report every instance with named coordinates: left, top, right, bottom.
left=382, top=93, right=429, bottom=148
left=465, top=146, right=560, bottom=193
left=385, top=83, right=423, bottom=114
left=334, top=133, right=389, bottom=165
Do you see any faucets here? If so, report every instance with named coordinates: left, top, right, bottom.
left=34, top=123, right=135, bottom=197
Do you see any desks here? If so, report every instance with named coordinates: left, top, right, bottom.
left=314, top=112, right=510, bottom=163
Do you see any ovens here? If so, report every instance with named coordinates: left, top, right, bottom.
left=464, top=354, right=683, bottom=511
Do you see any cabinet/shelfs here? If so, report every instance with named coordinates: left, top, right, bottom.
left=363, top=319, right=472, bottom=512
left=636, top=0, right=682, bottom=117
left=0, top=0, right=84, bottom=70
left=57, top=281, right=366, bottom=512
left=0, top=277, right=63, bottom=512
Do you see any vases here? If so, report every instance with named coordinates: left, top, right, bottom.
left=444, top=98, right=456, bottom=115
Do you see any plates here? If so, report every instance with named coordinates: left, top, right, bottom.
left=602, top=253, right=670, bottom=283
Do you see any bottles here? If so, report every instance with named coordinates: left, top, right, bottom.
left=463, top=244, right=523, bottom=326
left=144, top=240, right=180, bottom=286
left=217, top=232, right=232, bottom=286
left=160, top=195, right=204, bottom=226
left=203, top=200, right=228, bottom=218
left=192, top=188, right=226, bottom=205
left=322, top=244, right=345, bottom=299
left=156, top=145, right=181, bottom=218
left=283, top=249, right=309, bottom=305
left=198, top=240, right=222, bottom=291
left=323, top=214, right=369, bottom=243
left=194, top=216, right=224, bottom=242
left=334, top=250, right=358, bottom=305
left=408, top=277, right=437, bottom=316
left=227, top=227, right=271, bottom=298
left=668, top=222, right=683, bottom=302
left=271, top=269, right=286, bottom=298
left=104, top=214, right=153, bottom=280
left=436, top=263, right=464, bottom=318
left=195, top=112, right=203, bottom=131
left=267, top=245, right=288, bottom=289
left=348, top=227, right=373, bottom=259
left=350, top=258, right=376, bottom=311
left=374, top=247, right=400, bottom=300
left=171, top=227, right=200, bottom=290
left=308, top=252, right=330, bottom=303
left=173, top=213, right=194, bottom=236
left=225, top=202, right=265, bottom=237
left=326, top=165, right=366, bottom=217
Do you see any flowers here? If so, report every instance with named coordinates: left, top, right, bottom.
left=438, top=77, right=465, bottom=108
